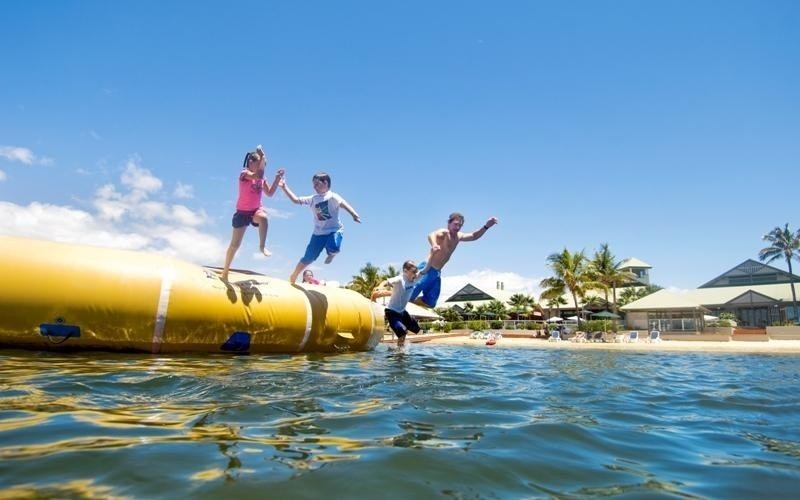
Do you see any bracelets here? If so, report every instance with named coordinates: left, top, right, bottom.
left=484, top=225, right=489, bottom=230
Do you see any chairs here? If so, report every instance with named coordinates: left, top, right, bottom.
left=547, top=331, right=561, bottom=342
left=627, top=331, right=639, bottom=342
left=646, top=331, right=663, bottom=343
left=568, top=331, right=622, bottom=343
left=470, top=331, right=502, bottom=340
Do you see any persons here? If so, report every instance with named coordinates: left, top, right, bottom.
left=372, top=260, right=421, bottom=351
left=370, top=211, right=499, bottom=310
left=302, top=269, right=320, bottom=284
left=274, top=172, right=362, bottom=285
left=220, top=144, right=284, bottom=283
